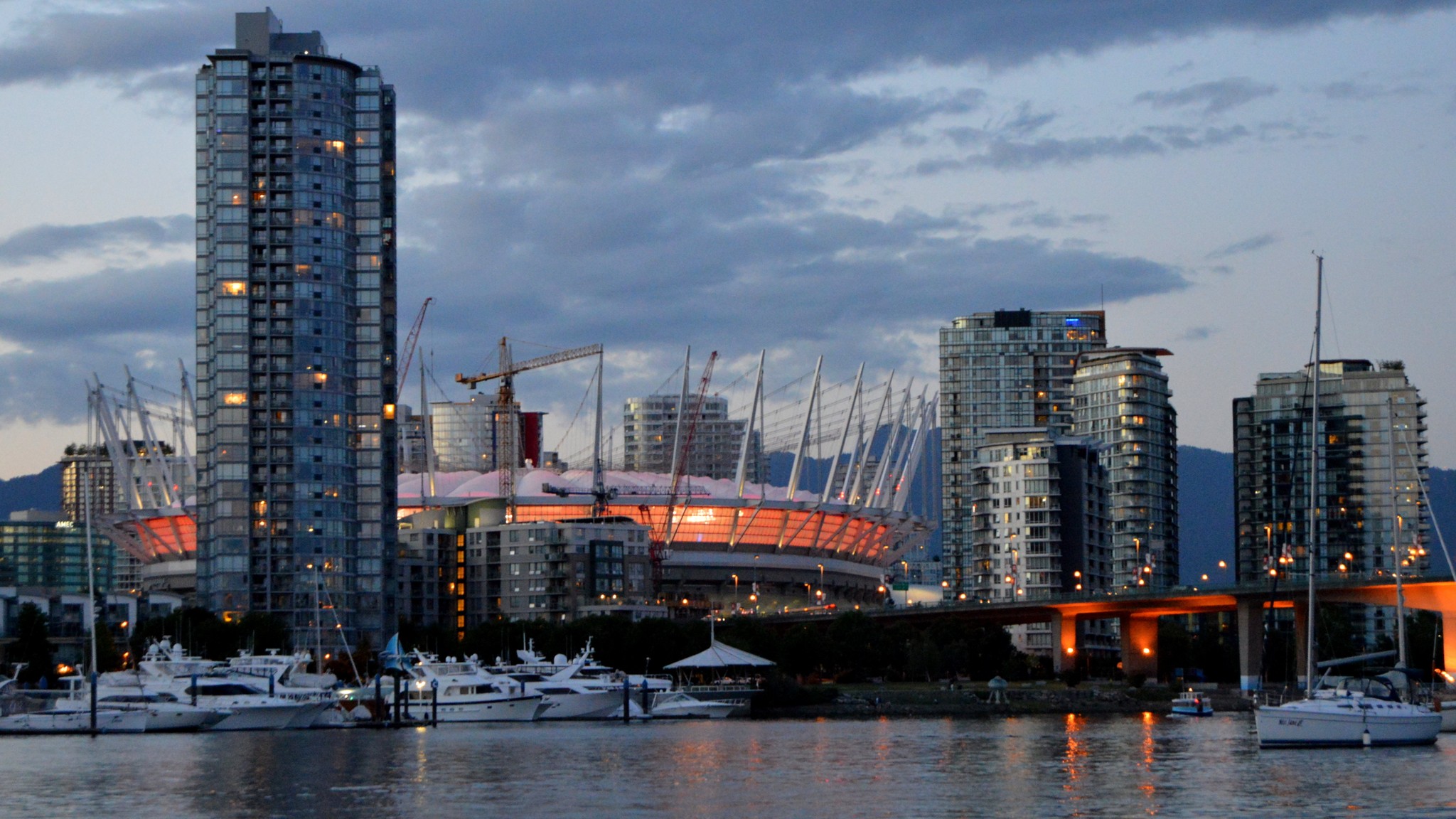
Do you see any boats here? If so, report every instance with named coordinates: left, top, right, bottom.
left=1172, top=688, right=1214, bottom=715
left=0, top=472, right=764, bottom=733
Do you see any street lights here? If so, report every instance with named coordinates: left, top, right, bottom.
left=804, top=564, right=824, bottom=612
left=1133, top=538, right=1152, bottom=587
left=943, top=581, right=953, bottom=600
left=1073, top=571, right=1082, bottom=594
left=1345, top=553, right=1353, bottom=573
left=1398, top=514, right=1425, bottom=576
left=1005, top=533, right=1022, bottom=601
left=1264, top=527, right=1293, bottom=580
left=731, top=555, right=759, bottom=612
left=879, top=561, right=908, bottom=605
left=1202, top=560, right=1227, bottom=585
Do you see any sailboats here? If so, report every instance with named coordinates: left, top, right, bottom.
left=1254, top=250, right=1456, bottom=745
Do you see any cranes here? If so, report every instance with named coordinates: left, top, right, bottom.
left=397, top=297, right=620, bottom=518
left=638, top=350, right=719, bottom=591
